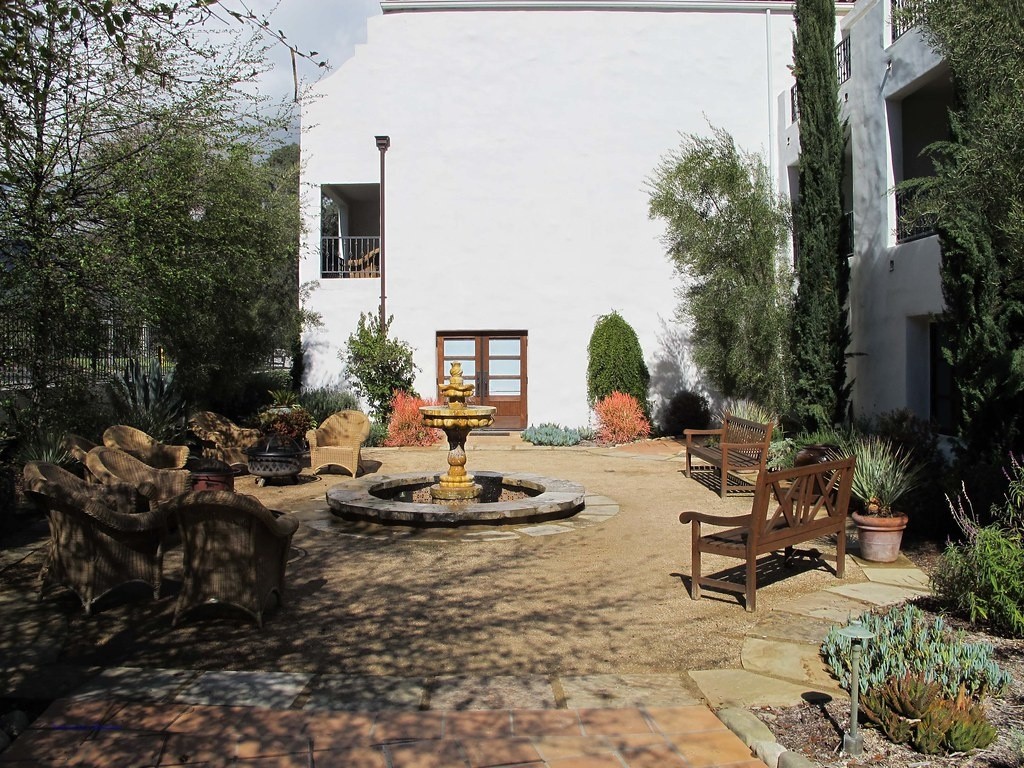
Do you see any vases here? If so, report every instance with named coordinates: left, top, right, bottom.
left=241, top=447, right=309, bottom=487
left=190, top=465, right=241, bottom=492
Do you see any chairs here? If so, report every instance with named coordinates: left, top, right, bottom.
left=17, top=410, right=299, bottom=641
left=306, top=409, right=371, bottom=479
left=347, top=248, right=378, bottom=278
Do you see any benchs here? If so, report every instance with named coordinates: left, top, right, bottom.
left=679, top=453, right=856, bottom=613
left=684, top=409, right=775, bottom=497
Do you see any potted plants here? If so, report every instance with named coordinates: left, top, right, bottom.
left=267, top=389, right=300, bottom=408
left=819, top=423, right=928, bottom=563
